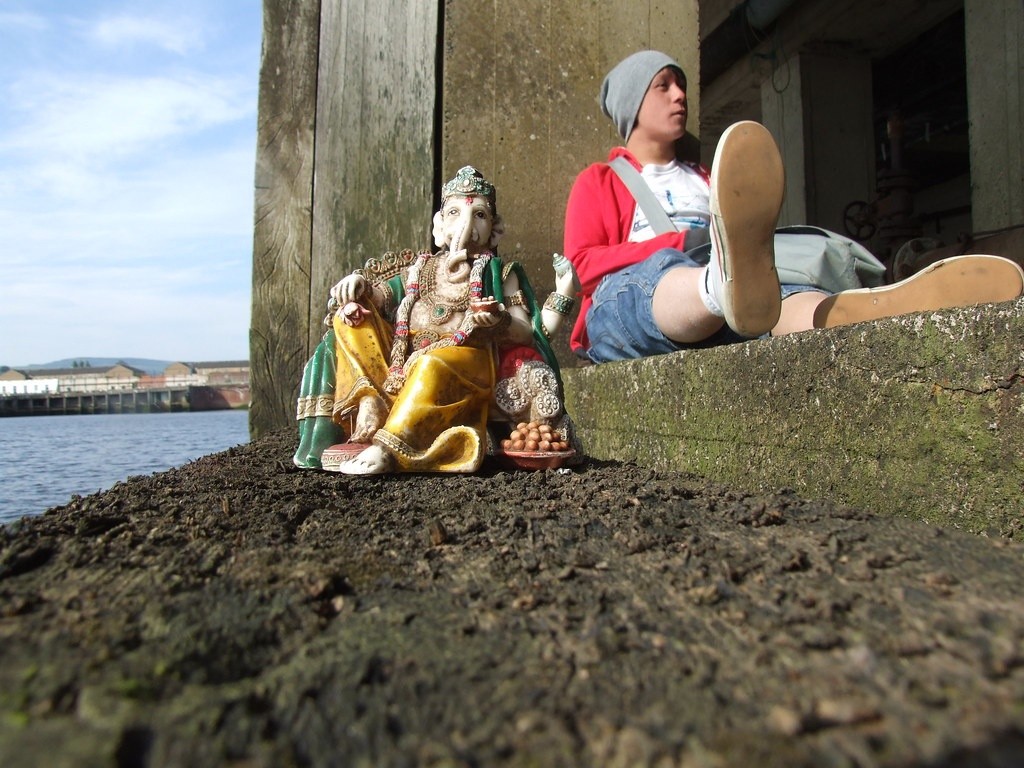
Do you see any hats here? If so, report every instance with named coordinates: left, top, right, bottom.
left=599, top=50, right=687, bottom=144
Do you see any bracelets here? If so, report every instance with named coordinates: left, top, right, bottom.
left=487, top=311, right=512, bottom=335
left=543, top=292, right=575, bottom=316
left=358, top=275, right=373, bottom=298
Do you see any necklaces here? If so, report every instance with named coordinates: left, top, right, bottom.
left=381, top=249, right=494, bottom=393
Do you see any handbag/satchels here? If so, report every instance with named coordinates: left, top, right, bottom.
left=772, top=225, right=886, bottom=294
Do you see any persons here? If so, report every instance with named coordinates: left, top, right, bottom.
left=563, top=51, right=1023, bottom=363
left=293, top=166, right=576, bottom=475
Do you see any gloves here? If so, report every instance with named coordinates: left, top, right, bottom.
left=684, top=227, right=711, bottom=263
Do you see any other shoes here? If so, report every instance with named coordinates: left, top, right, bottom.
left=704, top=120, right=785, bottom=338
left=813, top=254, right=1024, bottom=328
